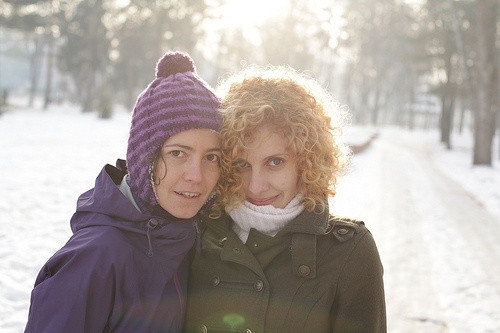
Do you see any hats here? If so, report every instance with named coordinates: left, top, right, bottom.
left=124, top=50, right=223, bottom=216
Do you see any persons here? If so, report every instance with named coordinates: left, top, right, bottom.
left=23, top=52, right=224, bottom=333
left=184, top=66, right=387, bottom=333
